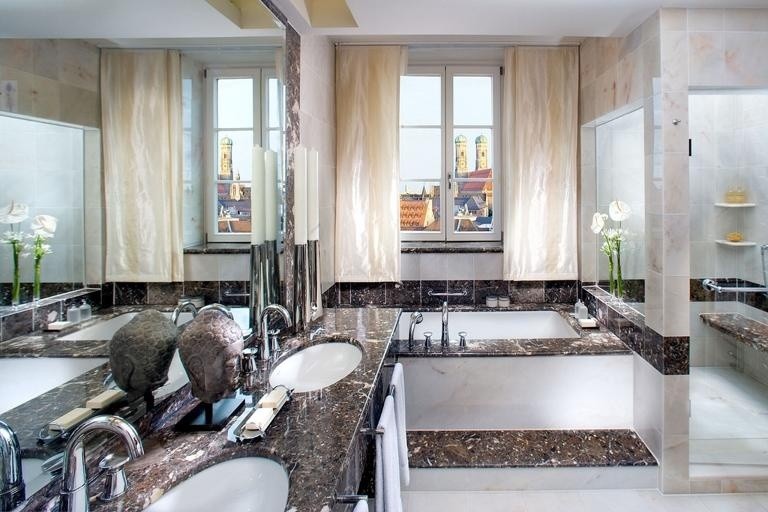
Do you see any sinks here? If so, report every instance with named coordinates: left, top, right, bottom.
left=19, top=449, right=52, bottom=495
left=128, top=444, right=290, bottom=512
left=268, top=337, right=363, bottom=393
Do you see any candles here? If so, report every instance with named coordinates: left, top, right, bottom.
left=294, top=144, right=320, bottom=246
left=250, top=143, right=278, bottom=246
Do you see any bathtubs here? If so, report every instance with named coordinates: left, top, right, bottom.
left=398, top=307, right=582, bottom=340
left=52, top=308, right=251, bottom=341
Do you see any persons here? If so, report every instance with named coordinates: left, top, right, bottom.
left=108, top=310, right=181, bottom=394
left=179, top=309, right=245, bottom=406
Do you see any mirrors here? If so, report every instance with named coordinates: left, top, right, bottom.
left=1, top=2, right=299, bottom=510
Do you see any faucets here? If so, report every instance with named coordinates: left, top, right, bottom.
left=171, top=302, right=197, bottom=325
left=0, top=421, right=25, bottom=512
left=441, top=301, right=449, bottom=347
left=60, top=415, right=144, bottom=512
left=255, top=304, right=292, bottom=359
left=198, top=304, right=234, bottom=326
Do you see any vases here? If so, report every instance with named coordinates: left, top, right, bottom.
left=609, top=266, right=624, bottom=304
left=11, top=269, right=41, bottom=311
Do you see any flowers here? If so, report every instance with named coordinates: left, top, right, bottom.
left=1, top=199, right=56, bottom=299
left=590, top=199, right=631, bottom=297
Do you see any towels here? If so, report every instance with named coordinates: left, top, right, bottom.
left=374, top=362, right=410, bottom=512
